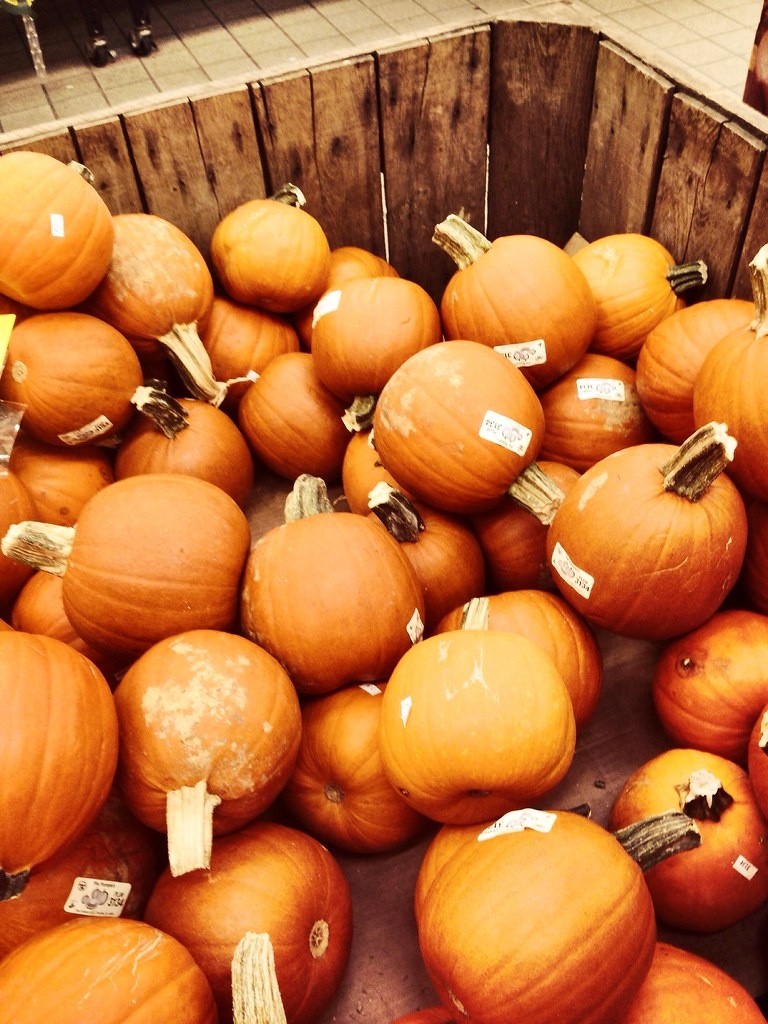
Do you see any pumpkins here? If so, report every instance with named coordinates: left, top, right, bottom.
left=1, top=150, right=768, bottom=1024
